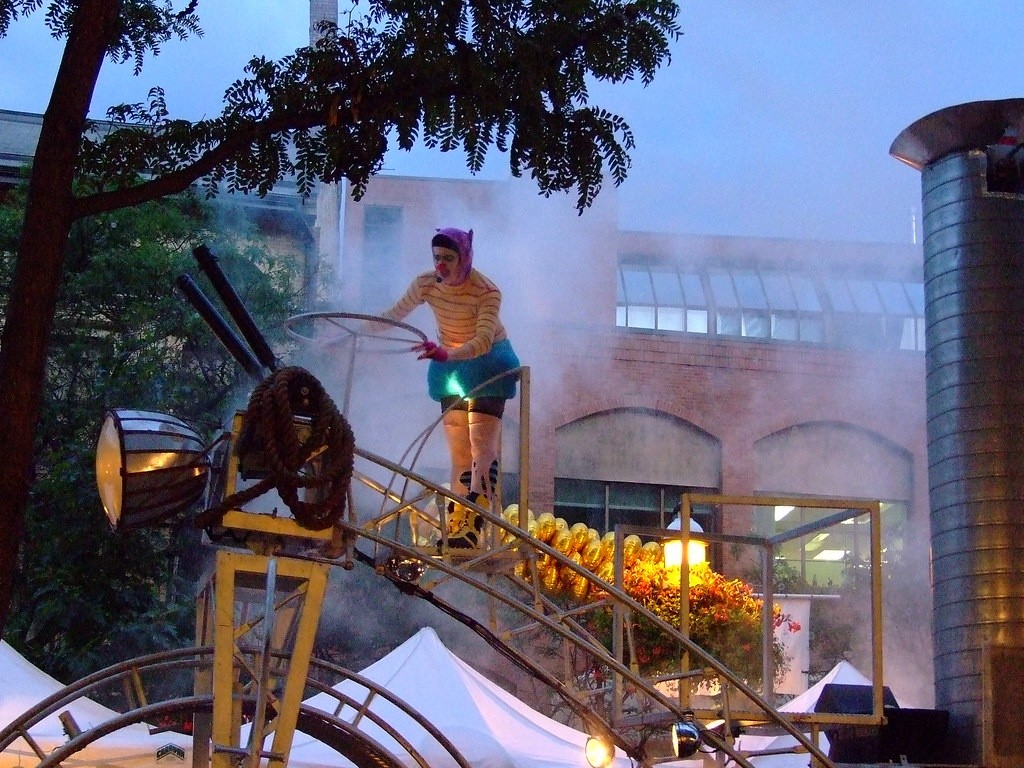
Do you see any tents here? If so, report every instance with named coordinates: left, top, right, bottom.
left=0, top=627, right=874, bottom=768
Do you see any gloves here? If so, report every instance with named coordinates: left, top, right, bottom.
left=411, top=340, right=448, bottom=362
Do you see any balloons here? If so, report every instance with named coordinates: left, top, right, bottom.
left=482, top=504, right=663, bottom=602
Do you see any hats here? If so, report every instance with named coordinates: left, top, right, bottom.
left=431, top=227, right=474, bottom=284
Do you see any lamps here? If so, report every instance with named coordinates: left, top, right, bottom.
left=94, top=407, right=214, bottom=532
left=584, top=734, right=615, bottom=768
left=672, top=722, right=725, bottom=758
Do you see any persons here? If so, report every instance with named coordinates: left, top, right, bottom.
left=358, top=225, right=522, bottom=547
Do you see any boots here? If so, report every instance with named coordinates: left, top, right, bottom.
left=436, top=492, right=490, bottom=548
